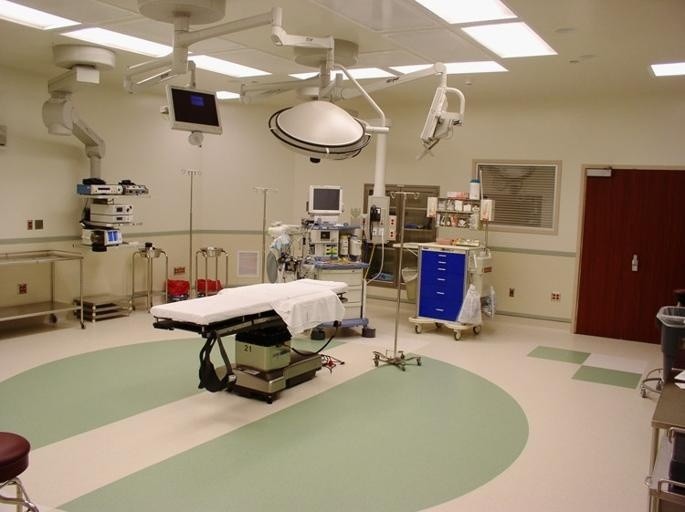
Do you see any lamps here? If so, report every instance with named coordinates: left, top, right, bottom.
left=266, top=36, right=390, bottom=164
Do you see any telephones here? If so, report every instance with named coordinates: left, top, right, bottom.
left=370, top=205, right=381, bottom=222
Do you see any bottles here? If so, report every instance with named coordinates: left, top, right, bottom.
left=470, top=179, right=480, bottom=200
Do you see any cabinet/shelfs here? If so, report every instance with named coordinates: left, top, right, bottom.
left=435, top=196, right=479, bottom=228
left=317, top=268, right=363, bottom=320
left=362, top=183, right=439, bottom=291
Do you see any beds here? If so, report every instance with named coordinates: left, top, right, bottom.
left=149, top=278, right=348, bottom=404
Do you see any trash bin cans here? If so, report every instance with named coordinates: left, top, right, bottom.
left=656, top=306, right=685, bottom=385
left=402, top=267, right=418, bottom=300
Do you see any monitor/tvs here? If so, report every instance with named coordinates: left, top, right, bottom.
left=165, top=84, right=223, bottom=135
left=309, top=184, right=343, bottom=223
